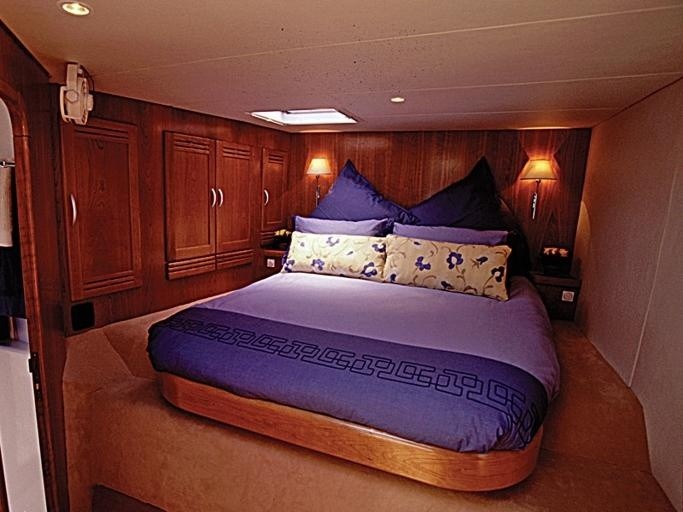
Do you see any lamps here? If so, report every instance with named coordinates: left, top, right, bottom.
left=520, top=155, right=560, bottom=221
left=306, top=157, right=332, bottom=208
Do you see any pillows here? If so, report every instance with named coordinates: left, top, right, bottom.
left=283, top=155, right=515, bottom=303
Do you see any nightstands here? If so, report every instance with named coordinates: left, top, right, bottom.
left=258, top=246, right=287, bottom=277
left=531, top=270, right=582, bottom=323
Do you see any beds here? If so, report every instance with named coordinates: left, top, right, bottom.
left=145, top=268, right=560, bottom=493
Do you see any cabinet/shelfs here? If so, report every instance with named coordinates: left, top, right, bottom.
left=165, top=130, right=261, bottom=280
left=48, top=115, right=146, bottom=303
left=262, top=148, right=293, bottom=246
